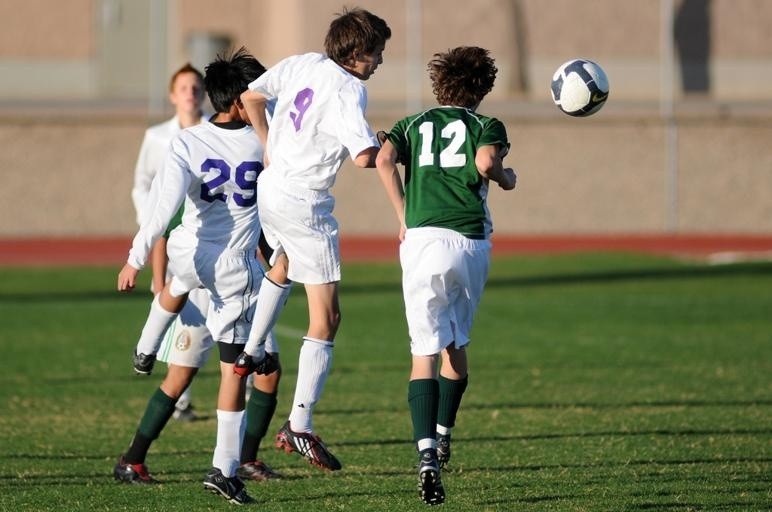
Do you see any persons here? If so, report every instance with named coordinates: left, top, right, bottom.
left=375, top=44, right=516, bottom=506
left=114, top=47, right=283, bottom=506
left=232, top=6, right=400, bottom=472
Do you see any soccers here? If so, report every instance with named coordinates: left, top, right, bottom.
left=551, top=58, right=609, bottom=117
